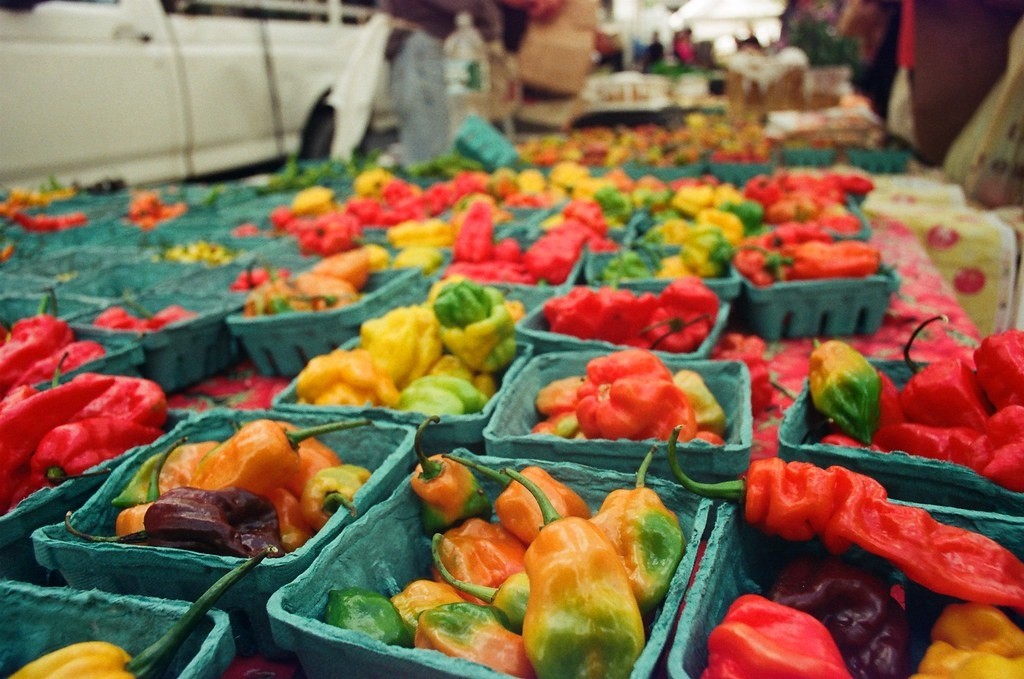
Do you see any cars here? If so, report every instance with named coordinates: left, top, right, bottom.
left=0, top=0, right=386, bottom=194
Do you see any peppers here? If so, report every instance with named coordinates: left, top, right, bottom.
left=0, top=104, right=1024, bottom=679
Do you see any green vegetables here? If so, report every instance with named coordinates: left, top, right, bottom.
left=648, top=61, right=713, bottom=81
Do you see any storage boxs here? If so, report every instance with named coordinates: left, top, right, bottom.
left=0, top=0, right=1024, bottom=679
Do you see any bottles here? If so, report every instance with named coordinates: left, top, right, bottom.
left=441, top=12, right=491, bottom=118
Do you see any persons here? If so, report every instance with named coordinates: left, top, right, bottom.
left=838, top=0, right=901, bottom=113
left=609, top=25, right=764, bottom=74
left=372, top=0, right=567, bottom=168
